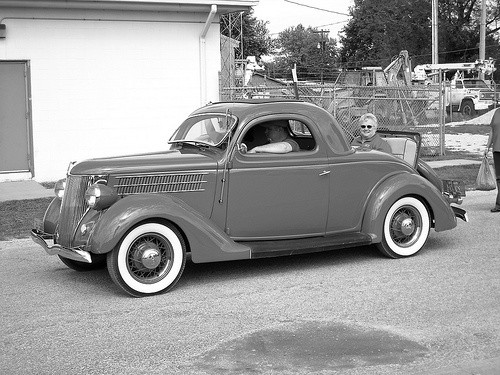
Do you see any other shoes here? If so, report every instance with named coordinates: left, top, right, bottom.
left=491, top=208, right=500, bottom=212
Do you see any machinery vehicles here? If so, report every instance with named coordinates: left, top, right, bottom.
left=408, top=57, right=500, bottom=119
left=322, top=50, right=416, bottom=124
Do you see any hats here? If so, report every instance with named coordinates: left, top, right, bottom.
left=260, top=120, right=289, bottom=127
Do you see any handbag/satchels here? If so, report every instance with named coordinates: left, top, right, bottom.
left=474, top=152, right=496, bottom=191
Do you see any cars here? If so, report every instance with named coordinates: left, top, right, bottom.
left=30, top=100, right=469, bottom=297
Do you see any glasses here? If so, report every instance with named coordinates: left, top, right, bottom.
left=361, top=125, right=375, bottom=129
